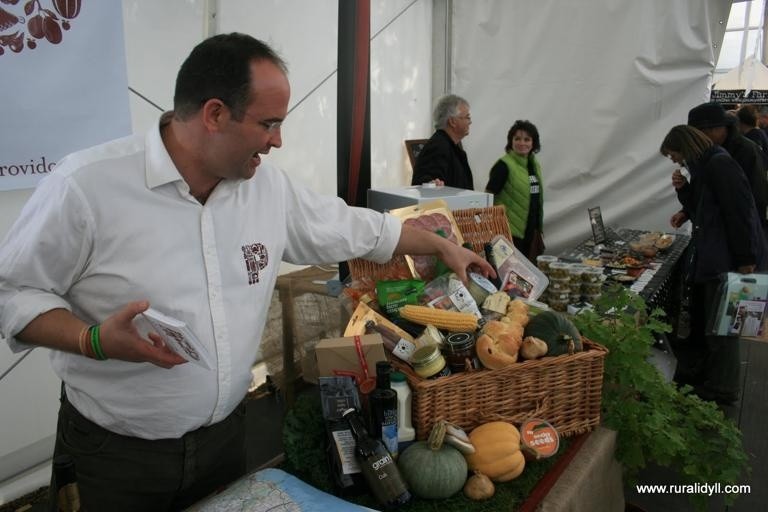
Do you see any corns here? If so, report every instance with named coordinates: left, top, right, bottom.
left=397, top=305, right=478, bottom=334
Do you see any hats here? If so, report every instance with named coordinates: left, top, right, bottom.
left=687, top=101, right=739, bottom=133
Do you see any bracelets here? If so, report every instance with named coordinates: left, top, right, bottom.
left=78, top=323, right=107, bottom=361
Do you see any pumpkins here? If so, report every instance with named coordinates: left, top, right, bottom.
left=521, top=336, right=549, bottom=361
left=524, top=311, right=583, bottom=357
left=464, top=422, right=539, bottom=484
left=397, top=422, right=468, bottom=500
left=463, top=470, right=495, bottom=501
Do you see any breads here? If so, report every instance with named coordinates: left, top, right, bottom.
left=476, top=300, right=529, bottom=370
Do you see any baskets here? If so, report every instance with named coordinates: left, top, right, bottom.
left=343, top=204, right=613, bottom=456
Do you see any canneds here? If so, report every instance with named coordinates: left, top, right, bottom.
left=445, top=331, right=476, bottom=373
left=409, top=343, right=451, bottom=381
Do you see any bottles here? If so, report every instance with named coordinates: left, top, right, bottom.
left=342, top=361, right=414, bottom=510
left=484, top=242, right=503, bottom=290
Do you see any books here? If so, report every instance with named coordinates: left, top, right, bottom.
left=141, top=308, right=217, bottom=371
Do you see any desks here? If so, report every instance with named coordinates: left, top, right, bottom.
left=184, top=425, right=626, bottom=512
left=558, top=226, right=690, bottom=347
left=274, top=265, right=350, bottom=405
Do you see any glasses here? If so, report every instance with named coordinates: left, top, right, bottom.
left=235, top=111, right=284, bottom=135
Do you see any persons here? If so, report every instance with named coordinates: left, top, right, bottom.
left=660, top=100, right=768, bottom=405
left=411, top=95, right=475, bottom=191
left=484, top=119, right=547, bottom=269
left=0, top=29, right=498, bottom=512
